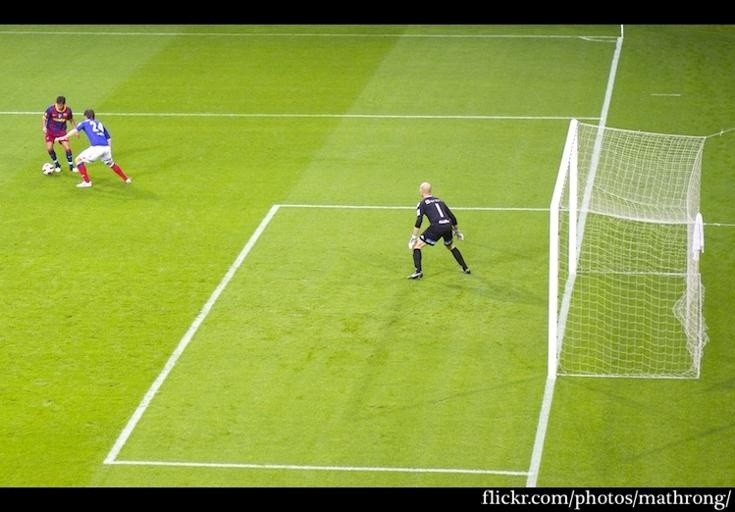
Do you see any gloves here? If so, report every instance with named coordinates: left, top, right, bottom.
left=408, top=234, right=418, bottom=249
left=454, top=229, right=464, bottom=241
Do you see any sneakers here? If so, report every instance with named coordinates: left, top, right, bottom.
left=68, top=162, right=75, bottom=171
left=76, top=180, right=92, bottom=187
left=461, top=269, right=471, bottom=274
left=407, top=272, right=423, bottom=279
left=54, top=161, right=61, bottom=172
left=121, top=178, right=131, bottom=183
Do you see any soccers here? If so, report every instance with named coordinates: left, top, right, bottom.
left=41, top=162, right=55, bottom=175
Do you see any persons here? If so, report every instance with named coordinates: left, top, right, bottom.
left=405, top=182, right=471, bottom=279
left=54, top=108, right=133, bottom=188
left=40, top=95, right=78, bottom=173
